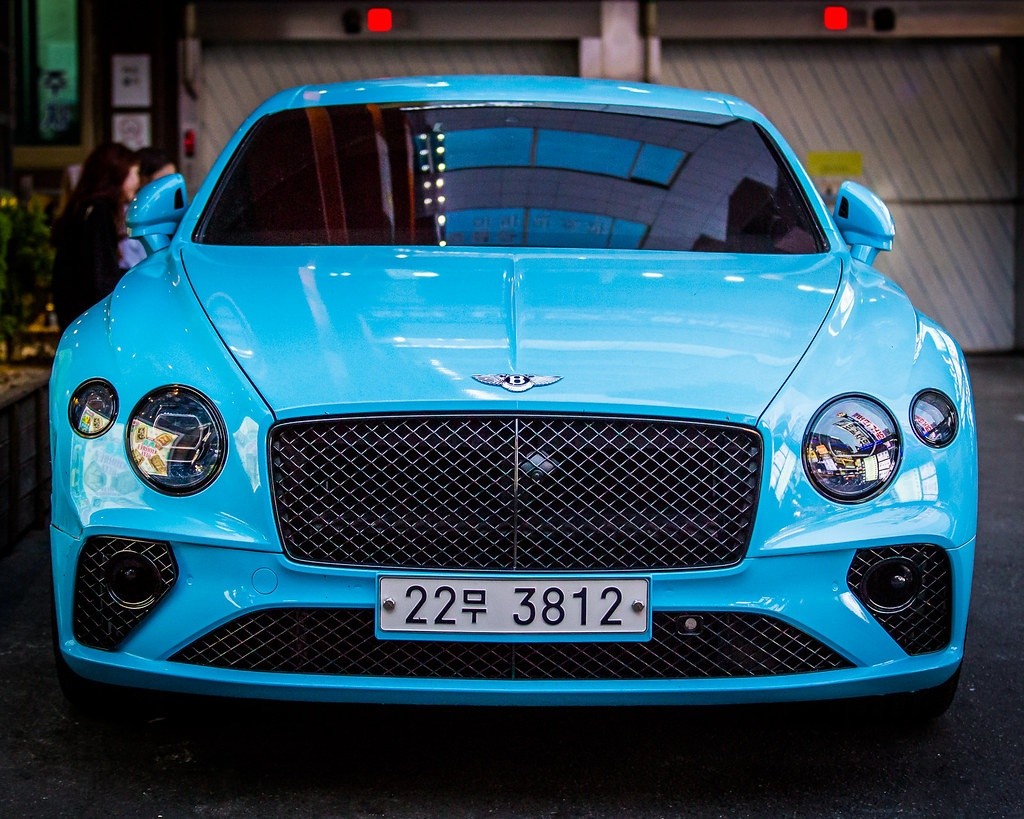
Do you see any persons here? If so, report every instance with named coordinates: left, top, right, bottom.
left=50, top=135, right=179, bottom=337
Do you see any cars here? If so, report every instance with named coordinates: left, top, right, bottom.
left=46, top=73, right=983, bottom=721
